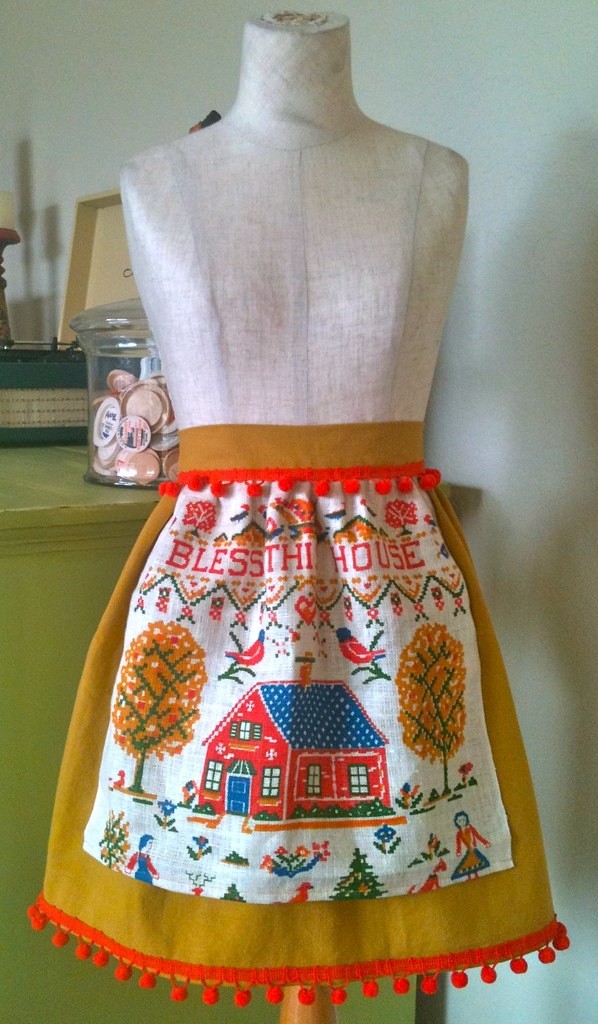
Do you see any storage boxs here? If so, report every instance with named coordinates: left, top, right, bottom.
left=0, top=346, right=140, bottom=449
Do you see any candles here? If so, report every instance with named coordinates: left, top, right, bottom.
left=0, top=190, right=16, bottom=231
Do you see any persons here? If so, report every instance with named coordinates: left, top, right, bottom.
left=25, top=12, right=571, bottom=1024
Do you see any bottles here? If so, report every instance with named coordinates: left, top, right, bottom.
left=70, top=293, right=183, bottom=490
